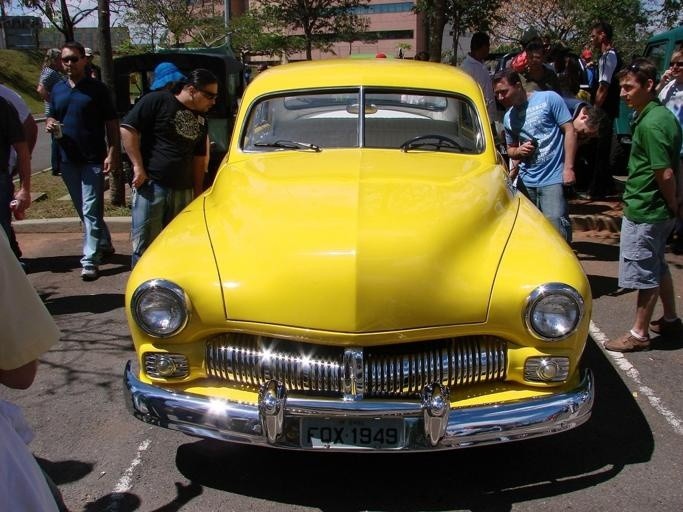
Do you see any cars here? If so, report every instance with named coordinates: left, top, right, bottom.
left=121, top=56, right=594, bottom=456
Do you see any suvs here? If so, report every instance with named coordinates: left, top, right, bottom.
left=608, top=26, right=683, bottom=178
left=109, top=43, right=242, bottom=207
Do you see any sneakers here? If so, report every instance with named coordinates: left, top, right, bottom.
left=604, top=330, right=652, bottom=354
left=649, top=318, right=683, bottom=334
left=80, top=267, right=100, bottom=281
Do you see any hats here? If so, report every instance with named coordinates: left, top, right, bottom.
left=582, top=49, right=593, bottom=59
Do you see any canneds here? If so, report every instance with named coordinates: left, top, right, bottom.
left=52, top=120, right=63, bottom=139
left=10, top=199, right=26, bottom=221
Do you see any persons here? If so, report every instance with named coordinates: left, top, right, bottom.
left=564, top=98, right=607, bottom=137
left=459, top=31, right=498, bottom=141
left=119, top=68, right=218, bottom=272
left=0, top=85, right=38, bottom=179
left=0, top=225, right=63, bottom=512
left=654, top=46, right=683, bottom=155
left=518, top=41, right=563, bottom=97
left=562, top=55, right=573, bottom=70
left=45, top=41, right=119, bottom=280
left=573, top=22, right=623, bottom=200
left=581, top=49, right=595, bottom=89
left=543, top=36, right=553, bottom=55
left=499, top=30, right=543, bottom=71
left=0, top=97, right=31, bottom=272
left=492, top=69, right=578, bottom=243
left=604, top=59, right=683, bottom=353
left=37, top=48, right=62, bottom=176
left=84, top=48, right=101, bottom=82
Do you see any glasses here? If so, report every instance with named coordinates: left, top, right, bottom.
left=619, top=62, right=650, bottom=76
left=200, top=89, right=219, bottom=101
left=669, top=62, right=683, bottom=67
left=61, top=56, right=80, bottom=63
